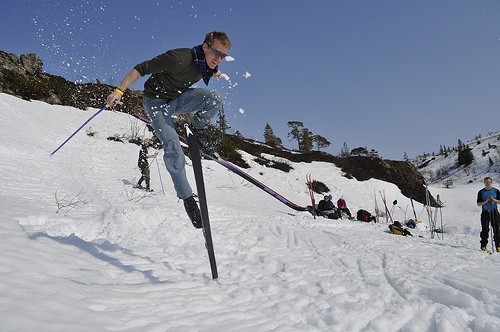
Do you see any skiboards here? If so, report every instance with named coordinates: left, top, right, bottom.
left=133, top=112, right=314, bottom=280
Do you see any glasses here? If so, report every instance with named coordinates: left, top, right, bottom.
left=484, top=181, right=491, bottom=184
left=208, top=44, right=227, bottom=60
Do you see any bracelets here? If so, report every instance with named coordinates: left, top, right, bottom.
left=115, top=88, right=124, bottom=96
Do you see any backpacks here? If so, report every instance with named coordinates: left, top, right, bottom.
left=406, top=219, right=416, bottom=229
left=357, top=209, right=376, bottom=223
left=389, top=224, right=413, bottom=236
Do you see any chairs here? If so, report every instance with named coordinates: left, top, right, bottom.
left=308, top=204, right=343, bottom=221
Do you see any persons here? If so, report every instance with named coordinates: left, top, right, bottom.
left=476, top=177, right=500, bottom=255
left=137, top=142, right=155, bottom=192
left=336, top=194, right=352, bottom=219
left=389, top=220, right=410, bottom=236
left=318, top=196, right=341, bottom=219
left=106, top=31, right=231, bottom=229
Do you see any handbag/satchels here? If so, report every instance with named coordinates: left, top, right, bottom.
left=328, top=212, right=338, bottom=219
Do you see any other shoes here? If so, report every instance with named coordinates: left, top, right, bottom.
left=137, top=184, right=143, bottom=187
left=496, top=247, right=500, bottom=252
left=146, top=189, right=154, bottom=192
left=183, top=195, right=203, bottom=228
left=190, top=124, right=203, bottom=148
left=482, top=247, right=488, bottom=253
